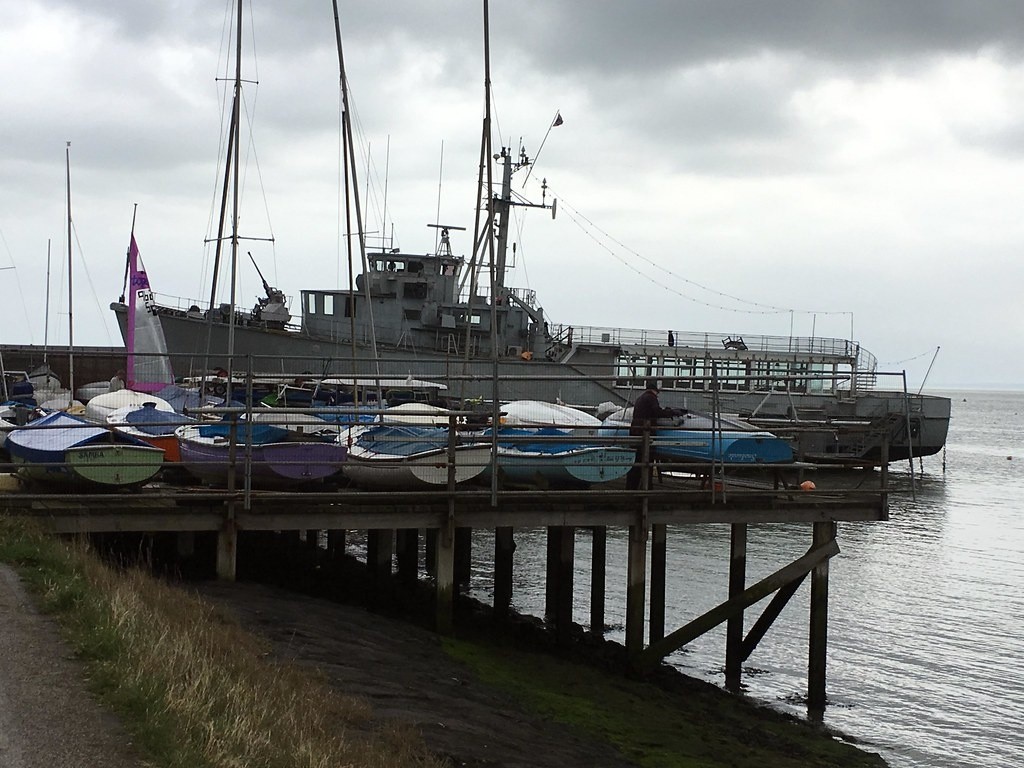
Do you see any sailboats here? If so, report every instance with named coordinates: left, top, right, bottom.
left=0, top=0, right=621, bottom=450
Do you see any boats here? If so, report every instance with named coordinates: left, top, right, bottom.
left=175, top=414, right=348, bottom=489
left=334, top=424, right=493, bottom=491
left=110, top=109, right=951, bottom=465
left=4, top=411, right=166, bottom=493
left=482, top=428, right=636, bottom=486
left=602, top=406, right=794, bottom=473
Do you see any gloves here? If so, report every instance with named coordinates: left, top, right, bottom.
left=671, top=407, right=684, bottom=416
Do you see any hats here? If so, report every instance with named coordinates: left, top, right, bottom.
left=646, top=378, right=663, bottom=393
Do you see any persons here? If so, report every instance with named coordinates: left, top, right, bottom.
left=106, top=369, right=125, bottom=393
left=624, top=378, right=690, bottom=495
